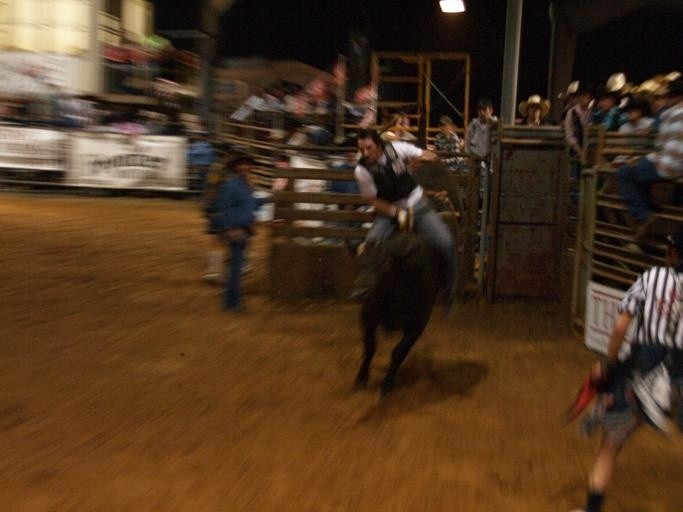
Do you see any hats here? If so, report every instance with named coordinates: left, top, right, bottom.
left=518, top=94, right=552, bottom=121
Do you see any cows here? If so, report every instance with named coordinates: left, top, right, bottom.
left=353, top=198, right=452, bottom=396
